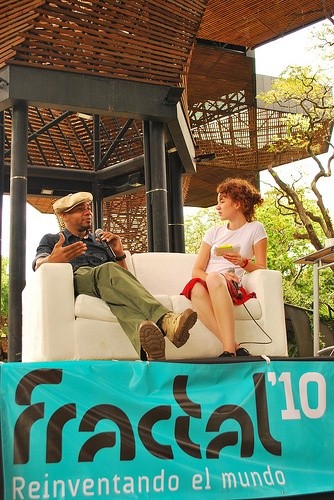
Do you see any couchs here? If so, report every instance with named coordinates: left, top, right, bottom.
left=22, top=252, right=290, bottom=361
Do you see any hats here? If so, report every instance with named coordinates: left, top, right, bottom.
left=52, top=192, right=94, bottom=215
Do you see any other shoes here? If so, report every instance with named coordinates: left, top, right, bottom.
left=236, top=347, right=251, bottom=356
left=217, top=351, right=235, bottom=358
left=158, top=307, right=198, bottom=348
left=138, top=321, right=165, bottom=360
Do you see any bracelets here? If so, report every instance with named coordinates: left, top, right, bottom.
left=241, top=259, right=248, bottom=268
left=115, top=254, right=126, bottom=261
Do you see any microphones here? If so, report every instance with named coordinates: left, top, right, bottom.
left=227, top=267, right=242, bottom=299
left=95, top=229, right=116, bottom=259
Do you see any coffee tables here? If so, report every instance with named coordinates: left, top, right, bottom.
left=294, top=246, right=334, bottom=357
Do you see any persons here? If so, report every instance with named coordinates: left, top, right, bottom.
left=185, top=177, right=269, bottom=360
left=33, top=190, right=199, bottom=362
